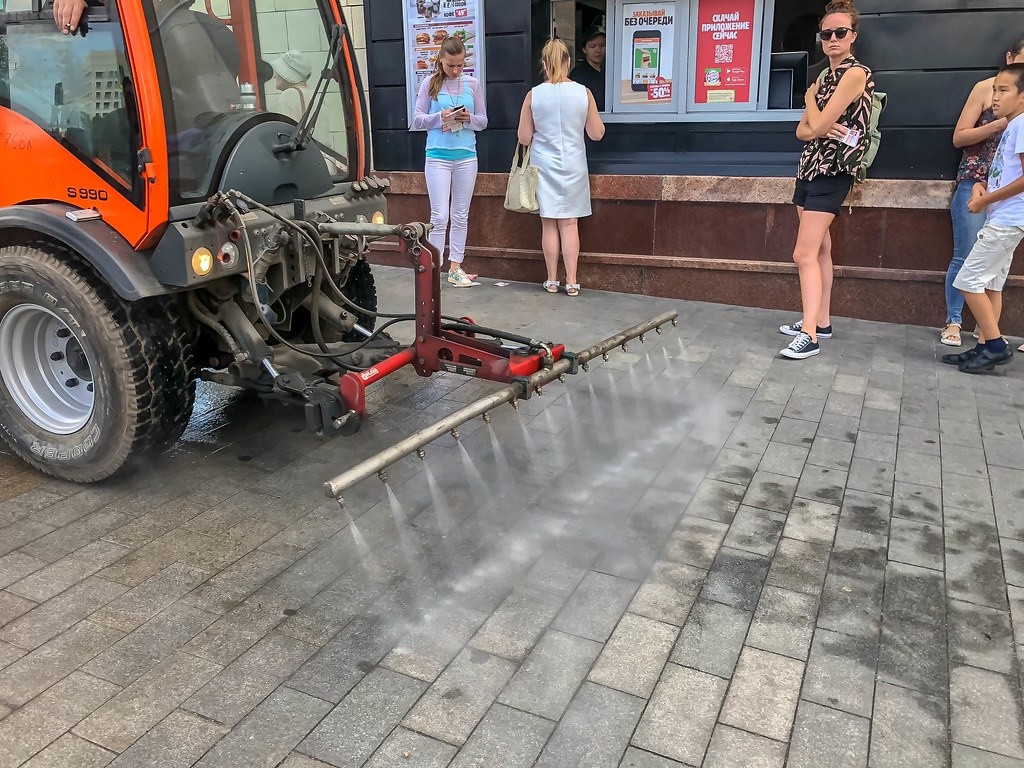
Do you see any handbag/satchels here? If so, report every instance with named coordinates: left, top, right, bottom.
left=504, top=141, right=539, bottom=213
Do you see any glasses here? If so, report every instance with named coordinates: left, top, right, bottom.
left=818, top=27, right=855, bottom=41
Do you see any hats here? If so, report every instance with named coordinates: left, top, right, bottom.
left=270, top=49, right=312, bottom=83
left=583, top=24, right=606, bottom=40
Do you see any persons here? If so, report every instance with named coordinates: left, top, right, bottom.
left=940, top=34, right=1024, bottom=346
left=517, top=38, right=606, bottom=296
left=941, top=61, right=1024, bottom=376
left=413, top=36, right=488, bottom=287
left=52, top=0, right=92, bottom=34
left=269, top=49, right=337, bottom=176
left=568, top=25, right=605, bottom=112
left=779, top=0, right=877, bottom=361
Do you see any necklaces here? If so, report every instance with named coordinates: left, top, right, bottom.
left=445, top=77, right=461, bottom=137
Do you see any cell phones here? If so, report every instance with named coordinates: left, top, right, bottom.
left=632, top=30, right=661, bottom=91
left=453, top=104, right=464, bottom=112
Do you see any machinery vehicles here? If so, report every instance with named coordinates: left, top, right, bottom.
left=0, top=0, right=685, bottom=514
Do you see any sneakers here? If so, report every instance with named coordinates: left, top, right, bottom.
left=448, top=267, right=473, bottom=288
left=780, top=317, right=832, bottom=339
left=780, top=330, right=821, bottom=359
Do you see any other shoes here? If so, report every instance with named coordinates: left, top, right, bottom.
left=1018, top=344, right=1024, bottom=352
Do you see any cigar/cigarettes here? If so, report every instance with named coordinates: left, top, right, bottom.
left=64, top=23, right=70, bottom=27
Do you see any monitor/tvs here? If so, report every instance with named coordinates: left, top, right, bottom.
left=768, top=51, right=808, bottom=112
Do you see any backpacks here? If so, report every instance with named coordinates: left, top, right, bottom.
left=819, top=65, right=888, bottom=184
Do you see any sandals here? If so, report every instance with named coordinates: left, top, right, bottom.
left=942, top=349, right=995, bottom=371
left=941, top=323, right=962, bottom=346
left=543, top=280, right=560, bottom=292
left=564, top=284, right=580, bottom=296
left=959, top=344, right=1013, bottom=373
left=972, top=324, right=1010, bottom=345
